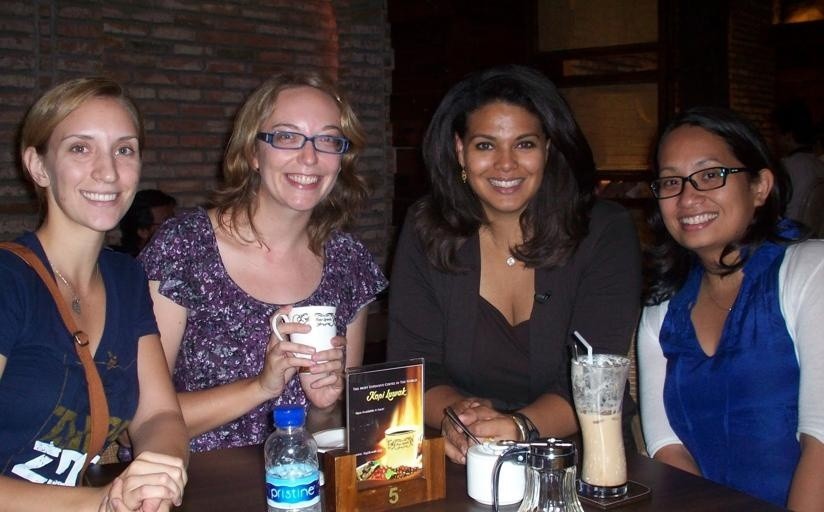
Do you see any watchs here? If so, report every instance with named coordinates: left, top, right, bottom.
left=525, top=413, right=539, bottom=443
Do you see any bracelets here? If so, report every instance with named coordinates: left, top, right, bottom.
left=509, top=414, right=529, bottom=441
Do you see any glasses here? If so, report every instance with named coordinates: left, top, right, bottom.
left=649, top=167, right=750, bottom=200
left=256, top=130, right=350, bottom=154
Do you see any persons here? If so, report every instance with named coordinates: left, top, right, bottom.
left=1, top=78, right=190, bottom=511
left=118, top=65, right=390, bottom=461
left=636, top=105, right=824, bottom=512
left=385, top=64, right=645, bottom=464
left=779, top=115, right=824, bottom=225
left=108, top=189, right=177, bottom=257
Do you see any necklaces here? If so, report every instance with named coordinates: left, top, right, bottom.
left=488, top=228, right=520, bottom=267
left=50, top=261, right=102, bottom=313
left=704, top=286, right=736, bottom=312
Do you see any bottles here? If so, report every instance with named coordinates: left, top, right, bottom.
left=264, top=406, right=320, bottom=511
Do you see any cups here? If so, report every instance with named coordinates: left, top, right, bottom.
left=467, top=444, right=526, bottom=505
left=271, top=307, right=338, bottom=363
left=381, top=423, right=419, bottom=463
left=574, top=356, right=630, bottom=498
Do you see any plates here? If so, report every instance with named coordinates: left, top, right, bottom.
left=310, top=427, right=350, bottom=454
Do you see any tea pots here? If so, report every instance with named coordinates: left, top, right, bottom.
left=491, top=435, right=583, bottom=512
